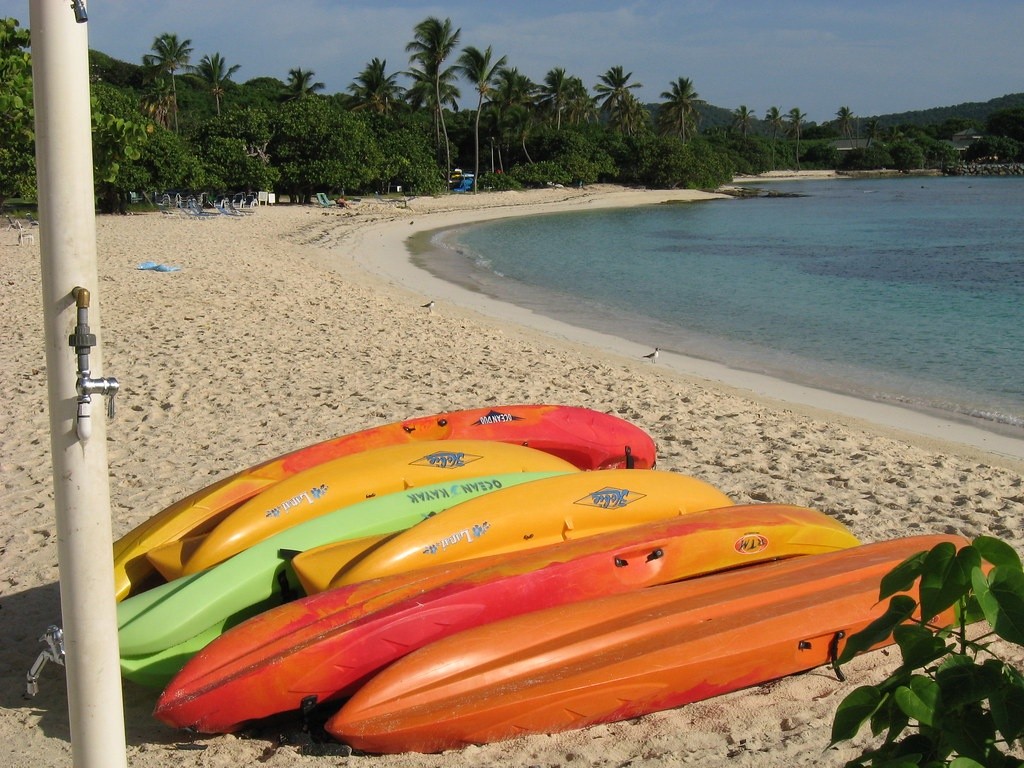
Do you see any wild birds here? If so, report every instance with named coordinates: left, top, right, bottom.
left=420, top=300, right=435, bottom=313
left=641, top=347, right=663, bottom=364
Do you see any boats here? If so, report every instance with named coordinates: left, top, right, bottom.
left=112, top=401, right=998, bottom=755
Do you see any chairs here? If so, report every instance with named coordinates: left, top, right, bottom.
left=6, top=211, right=39, bottom=246
left=314, top=192, right=419, bottom=218
left=160, top=191, right=257, bottom=219
